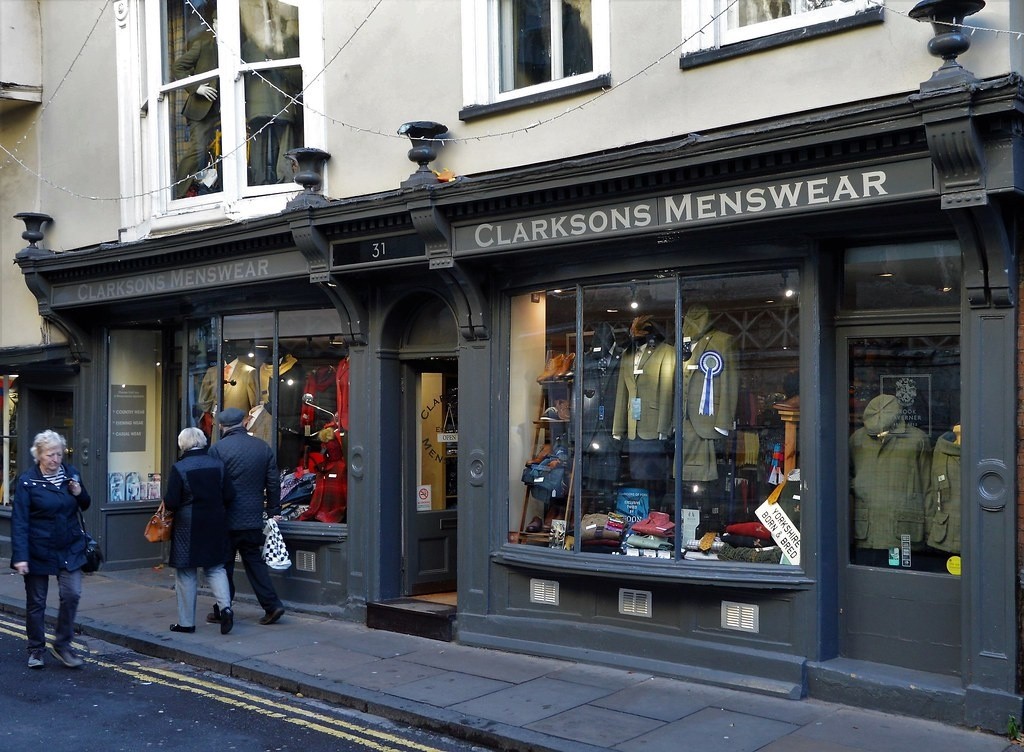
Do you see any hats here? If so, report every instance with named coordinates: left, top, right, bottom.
left=682, top=303, right=709, bottom=341
left=863, top=394, right=900, bottom=437
left=218, top=408, right=244, bottom=426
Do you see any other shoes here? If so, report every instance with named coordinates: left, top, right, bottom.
left=540, top=407, right=558, bottom=421
left=526, top=511, right=575, bottom=533
left=526, top=445, right=551, bottom=468
left=556, top=400, right=570, bottom=420
left=207, top=613, right=220, bottom=623
left=259, top=606, right=285, bottom=625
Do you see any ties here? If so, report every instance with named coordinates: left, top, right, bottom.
left=225, top=366, right=231, bottom=379
left=597, top=355, right=608, bottom=406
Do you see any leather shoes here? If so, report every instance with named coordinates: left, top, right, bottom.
left=536, top=354, right=566, bottom=383
left=553, top=353, right=576, bottom=380
left=220, top=607, right=234, bottom=634
left=170, top=624, right=195, bottom=633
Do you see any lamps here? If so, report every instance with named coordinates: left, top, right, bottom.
left=248, top=339, right=255, bottom=357
left=329, top=335, right=336, bottom=349
left=223, top=380, right=236, bottom=386
left=305, top=336, right=314, bottom=351
left=630, top=283, right=638, bottom=309
left=781, top=271, right=793, bottom=298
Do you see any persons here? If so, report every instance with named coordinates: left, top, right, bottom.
left=177, top=13, right=302, bottom=195
left=10, top=430, right=91, bottom=668
left=849, top=394, right=963, bottom=568
left=162, top=427, right=233, bottom=634
left=198, top=344, right=347, bottom=453
left=573, top=303, right=736, bottom=516
left=208, top=409, right=285, bottom=625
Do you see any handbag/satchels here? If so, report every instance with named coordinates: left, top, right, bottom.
left=78, top=505, right=104, bottom=572
left=261, top=519, right=292, bottom=570
left=145, top=500, right=173, bottom=543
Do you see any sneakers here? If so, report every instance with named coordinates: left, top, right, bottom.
left=52, top=646, right=84, bottom=666
left=28, top=649, right=44, bottom=667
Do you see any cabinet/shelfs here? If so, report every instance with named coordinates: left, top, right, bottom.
left=517, top=380, right=575, bottom=551
